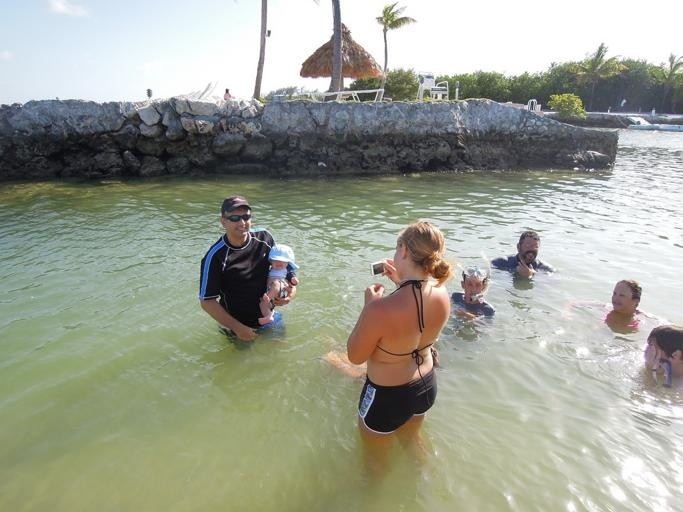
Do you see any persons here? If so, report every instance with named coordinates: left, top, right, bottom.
left=452, top=265, right=494, bottom=323
left=644, top=325, right=683, bottom=385
left=492, top=231, right=558, bottom=278
left=606, top=279, right=642, bottom=327
left=258, top=246, right=300, bottom=326
left=347, top=220, right=449, bottom=446
left=197, top=196, right=296, bottom=347
left=224, top=88, right=234, bottom=100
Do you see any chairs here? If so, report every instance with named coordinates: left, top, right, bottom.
left=417, top=71, right=449, bottom=100
left=198, top=81, right=219, bottom=100
left=527, top=99, right=538, bottom=111
left=300, top=89, right=385, bottom=102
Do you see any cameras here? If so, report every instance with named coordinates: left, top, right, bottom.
left=370, top=260, right=387, bottom=277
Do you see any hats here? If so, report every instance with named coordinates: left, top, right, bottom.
left=220, top=196, right=251, bottom=212
left=268, top=244, right=299, bottom=269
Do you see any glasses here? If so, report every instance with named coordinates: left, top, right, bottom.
left=462, top=265, right=488, bottom=282
left=221, top=214, right=251, bottom=222
